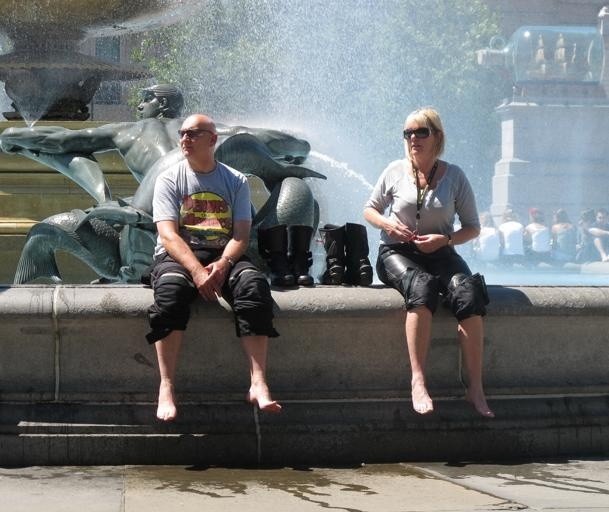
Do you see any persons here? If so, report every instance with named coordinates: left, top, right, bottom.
left=498, top=207, right=526, bottom=262
left=549, top=208, right=578, bottom=262
left=472, top=211, right=502, bottom=262
left=574, top=211, right=596, bottom=262
left=363, top=108, right=497, bottom=419
left=524, top=207, right=552, bottom=262
left=144, top=113, right=284, bottom=425
left=588, top=209, right=609, bottom=262
left=1, top=84, right=329, bottom=213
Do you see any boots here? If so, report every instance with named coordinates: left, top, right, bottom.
left=287, top=225, right=313, bottom=285
left=258, top=224, right=295, bottom=286
left=316, top=223, right=345, bottom=284
left=343, top=223, right=373, bottom=285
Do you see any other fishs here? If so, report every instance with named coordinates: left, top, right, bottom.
left=0, top=126, right=327, bottom=286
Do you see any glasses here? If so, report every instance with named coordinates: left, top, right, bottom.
left=180, top=130, right=213, bottom=139
left=404, top=128, right=429, bottom=139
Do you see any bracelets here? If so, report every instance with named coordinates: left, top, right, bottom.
left=226, top=256, right=237, bottom=267
